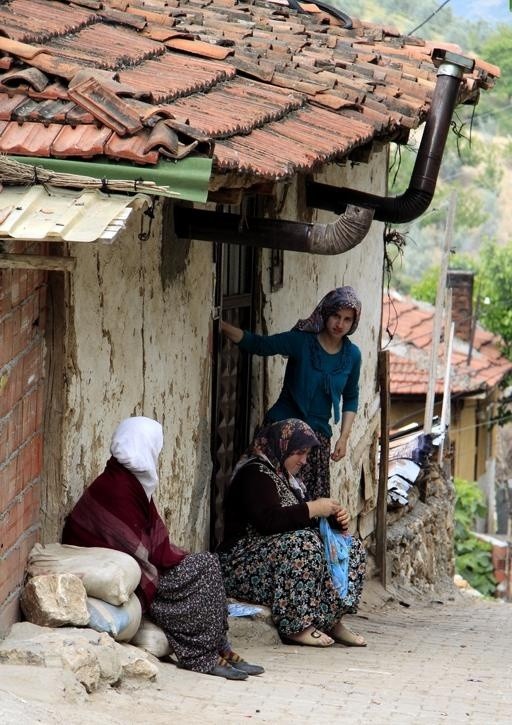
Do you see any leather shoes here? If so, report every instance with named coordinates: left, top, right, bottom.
left=225, top=655, right=265, bottom=676
left=208, top=664, right=248, bottom=680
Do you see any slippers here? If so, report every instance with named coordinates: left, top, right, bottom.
left=332, top=625, right=367, bottom=647
left=284, top=626, right=335, bottom=646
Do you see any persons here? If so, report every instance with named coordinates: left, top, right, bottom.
left=218, top=284, right=362, bottom=503
left=61, top=417, right=264, bottom=681
left=214, top=417, right=367, bottom=647
